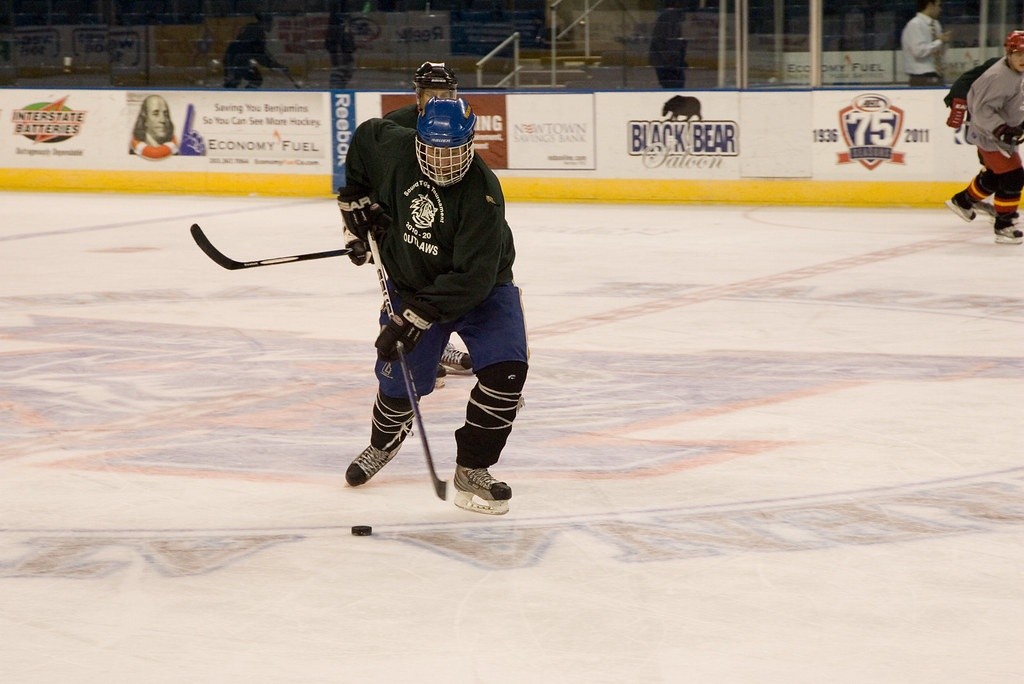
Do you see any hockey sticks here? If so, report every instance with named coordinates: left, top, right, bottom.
left=357, top=218, right=454, bottom=501
left=188, top=224, right=360, bottom=272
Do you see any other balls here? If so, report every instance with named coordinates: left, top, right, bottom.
left=350, top=525, right=373, bottom=537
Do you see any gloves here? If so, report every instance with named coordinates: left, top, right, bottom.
left=993, top=123, right=1024, bottom=145
left=342, top=226, right=374, bottom=267
left=376, top=301, right=437, bottom=363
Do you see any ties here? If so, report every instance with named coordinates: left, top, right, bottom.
left=931, top=23, right=938, bottom=64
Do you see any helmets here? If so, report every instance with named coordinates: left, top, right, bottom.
left=1004, top=30, right=1024, bottom=52
left=415, top=96, right=477, bottom=187
left=412, top=61, right=459, bottom=103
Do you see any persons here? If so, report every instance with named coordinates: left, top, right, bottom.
left=900, top=0, right=952, bottom=86
left=324, top=0, right=357, bottom=88
left=943, top=30, right=1024, bottom=244
left=338, top=97, right=529, bottom=515
left=343, top=61, right=474, bottom=388
left=221, top=12, right=289, bottom=87
left=128, top=95, right=179, bottom=156
left=647, top=4, right=689, bottom=88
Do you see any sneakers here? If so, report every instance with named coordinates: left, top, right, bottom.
left=344, top=442, right=403, bottom=488
left=442, top=343, right=475, bottom=376
left=945, top=191, right=977, bottom=223
left=994, top=223, right=1023, bottom=244
left=453, top=465, right=513, bottom=516
left=435, top=364, right=447, bottom=388
